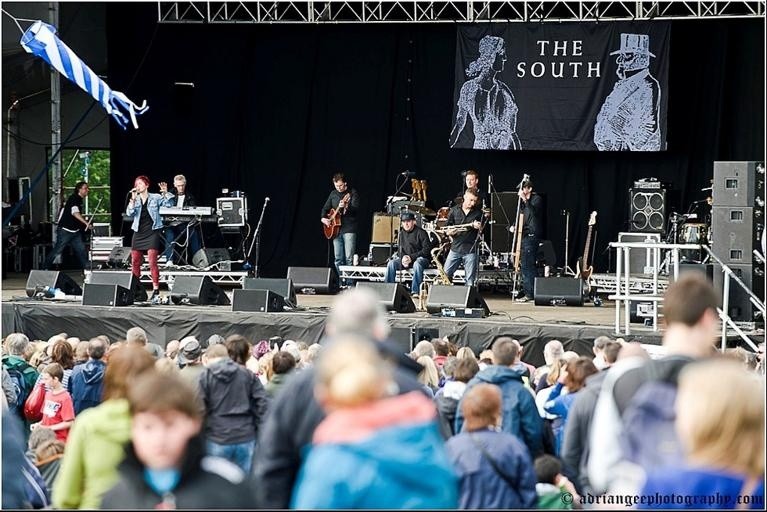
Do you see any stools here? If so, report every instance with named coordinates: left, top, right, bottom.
left=12, top=240, right=55, bottom=274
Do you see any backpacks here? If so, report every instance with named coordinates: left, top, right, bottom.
left=6, top=365, right=31, bottom=415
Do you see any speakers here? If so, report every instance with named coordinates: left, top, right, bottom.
left=628, top=187, right=667, bottom=235
left=425, top=285, right=491, bottom=317
left=711, top=160, right=764, bottom=206
left=713, top=264, right=764, bottom=322
left=534, top=276, right=584, bottom=307
left=107, top=246, right=144, bottom=267
left=669, top=264, right=713, bottom=286
left=24, top=266, right=340, bottom=311
left=355, top=281, right=416, bottom=313
left=711, top=206, right=765, bottom=264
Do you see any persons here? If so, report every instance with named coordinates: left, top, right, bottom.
left=0, top=272, right=767, bottom=512
left=52, top=183, right=95, bottom=270
left=125, top=175, right=174, bottom=296
left=706, top=194, right=712, bottom=208
left=384, top=213, right=431, bottom=299
left=449, top=34, right=523, bottom=150
left=454, top=171, right=484, bottom=211
left=592, top=32, right=662, bottom=152
left=165, top=175, right=201, bottom=268
left=320, top=173, right=361, bottom=286
left=509, top=179, right=541, bottom=303
left=443, top=189, right=486, bottom=286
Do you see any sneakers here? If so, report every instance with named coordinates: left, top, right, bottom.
left=515, top=296, right=534, bottom=303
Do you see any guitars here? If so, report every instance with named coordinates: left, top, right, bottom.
left=323, top=193, right=352, bottom=240
left=575, top=211, right=598, bottom=296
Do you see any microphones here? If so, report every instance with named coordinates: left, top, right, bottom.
left=625, top=219, right=642, bottom=225
left=192, top=248, right=231, bottom=267
left=129, top=188, right=136, bottom=193
left=488, top=175, right=491, bottom=194
left=402, top=170, right=416, bottom=176
left=695, top=199, right=711, bottom=204
left=263, top=197, right=270, bottom=206
left=516, top=174, right=530, bottom=188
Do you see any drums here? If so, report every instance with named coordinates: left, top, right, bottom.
left=682, top=223, right=708, bottom=244
left=436, top=207, right=454, bottom=226
left=422, top=228, right=453, bottom=268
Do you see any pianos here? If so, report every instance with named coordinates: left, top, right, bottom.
left=122, top=207, right=218, bottom=222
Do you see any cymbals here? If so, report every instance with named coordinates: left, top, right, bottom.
left=408, top=204, right=437, bottom=216
left=702, top=186, right=712, bottom=191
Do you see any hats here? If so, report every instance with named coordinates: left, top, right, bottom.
left=462, top=384, right=501, bottom=431
left=177, top=341, right=200, bottom=365
left=523, top=181, right=534, bottom=187
left=609, top=33, right=656, bottom=61
left=401, top=214, right=416, bottom=221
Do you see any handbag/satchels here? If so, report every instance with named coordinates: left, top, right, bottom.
left=23, top=384, right=45, bottom=419
